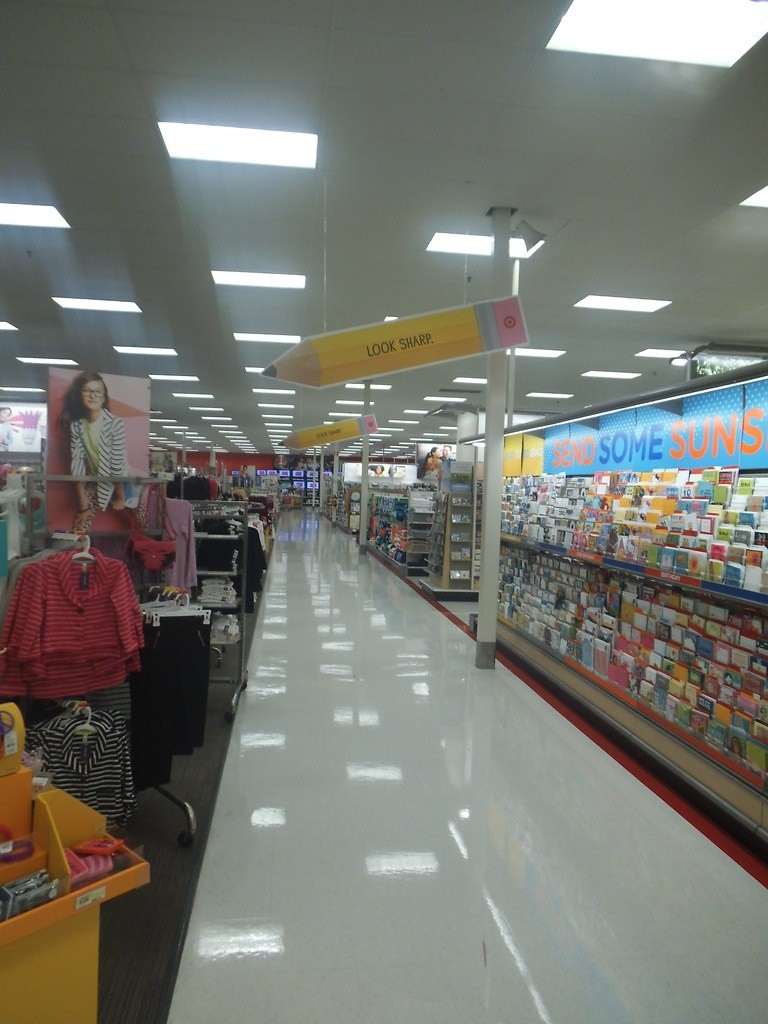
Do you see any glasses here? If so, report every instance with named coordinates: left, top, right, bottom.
left=80, top=389, right=105, bottom=397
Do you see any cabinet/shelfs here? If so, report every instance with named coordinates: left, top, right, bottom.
left=474, top=491, right=482, bottom=589
left=424, top=493, right=471, bottom=590
left=406, top=489, right=434, bottom=568
left=4, top=470, right=181, bottom=596
left=496, top=532, right=768, bottom=869
left=187, top=499, right=248, bottom=712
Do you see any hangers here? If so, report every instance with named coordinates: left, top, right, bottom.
left=49, top=532, right=94, bottom=561
left=140, top=586, right=212, bottom=626
left=56, top=699, right=98, bottom=736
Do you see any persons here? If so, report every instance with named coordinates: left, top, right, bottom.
left=307, top=457, right=320, bottom=470
left=388, top=465, right=406, bottom=478
left=242, top=465, right=248, bottom=477
left=438, top=445, right=455, bottom=462
left=368, top=465, right=384, bottom=477
left=162, top=454, right=173, bottom=473
left=0, top=407, right=14, bottom=451
left=53, top=371, right=149, bottom=531
left=292, top=457, right=305, bottom=470
left=149, top=454, right=153, bottom=471
left=422, top=447, right=443, bottom=479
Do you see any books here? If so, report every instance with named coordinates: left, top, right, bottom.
left=475, top=466, right=768, bottom=773
left=427, top=490, right=473, bottom=580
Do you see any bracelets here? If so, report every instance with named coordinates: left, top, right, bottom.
left=77, top=505, right=89, bottom=513
